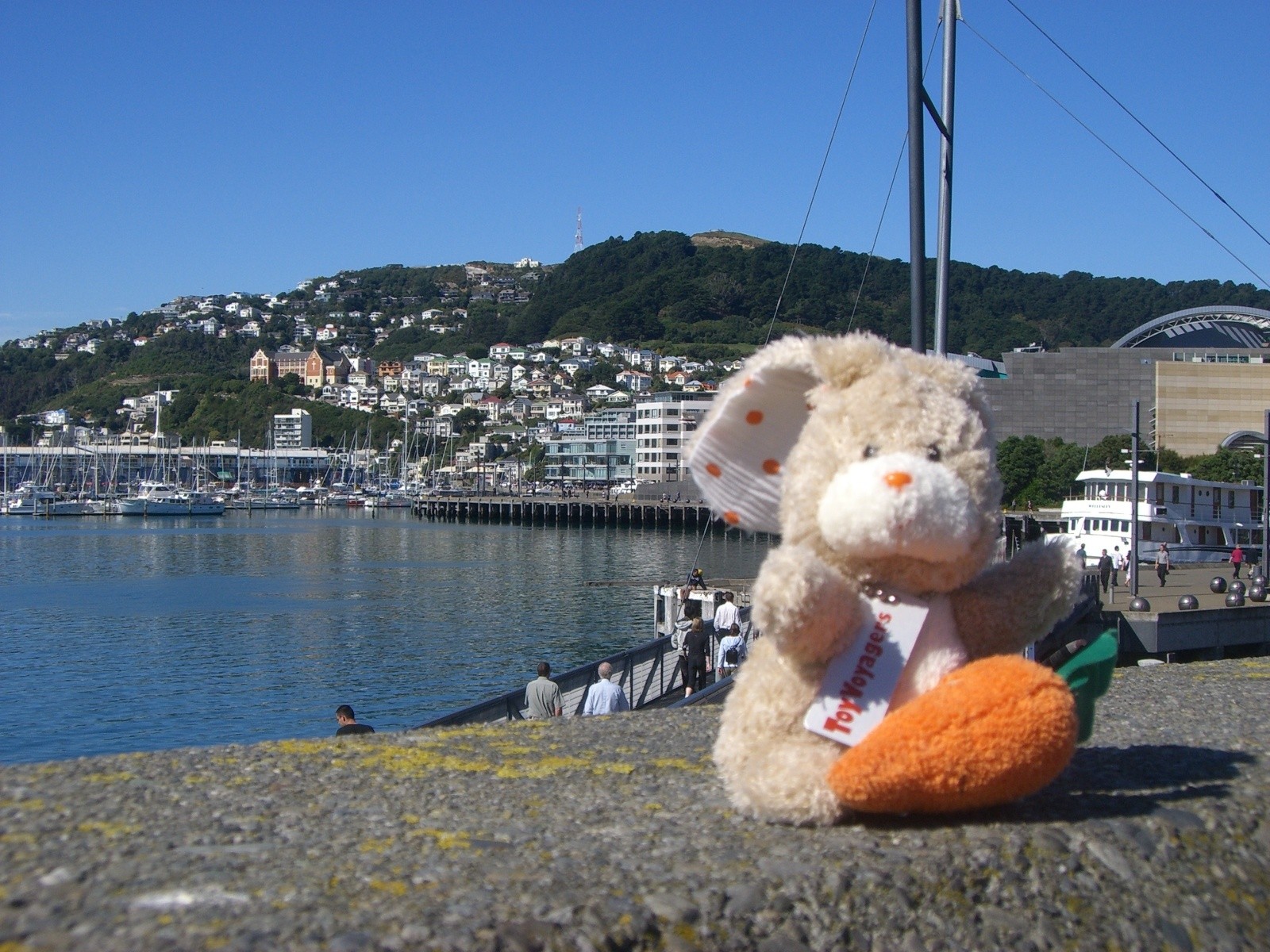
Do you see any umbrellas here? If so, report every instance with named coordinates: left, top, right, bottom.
left=574, top=480, right=583, bottom=483
left=563, top=480, right=572, bottom=483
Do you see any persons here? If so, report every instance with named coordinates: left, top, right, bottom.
left=615, top=493, right=618, bottom=502
left=334, top=705, right=376, bottom=736
left=687, top=568, right=707, bottom=590
left=584, top=661, right=629, bottom=716
left=1077, top=542, right=1086, bottom=565
left=674, top=591, right=751, bottom=700
left=1227, top=545, right=1259, bottom=580
left=524, top=662, right=566, bottom=719
left=493, top=487, right=496, bottom=497
left=1155, top=541, right=1170, bottom=588
left=585, top=491, right=589, bottom=498
left=1011, top=499, right=1016, bottom=514
left=1095, top=546, right=1132, bottom=594
left=568, top=489, right=572, bottom=498
left=563, top=488, right=567, bottom=499
left=1027, top=500, right=1032, bottom=512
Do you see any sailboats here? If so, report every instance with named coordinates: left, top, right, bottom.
left=0, top=376, right=538, bottom=518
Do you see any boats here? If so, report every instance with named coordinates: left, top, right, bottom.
left=1042, top=463, right=1268, bottom=567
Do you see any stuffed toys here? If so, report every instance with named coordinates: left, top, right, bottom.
left=682, top=328, right=1085, bottom=827
left=827, top=630, right=1120, bottom=817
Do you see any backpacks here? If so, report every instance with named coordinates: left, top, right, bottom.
left=726, top=638, right=741, bottom=664
